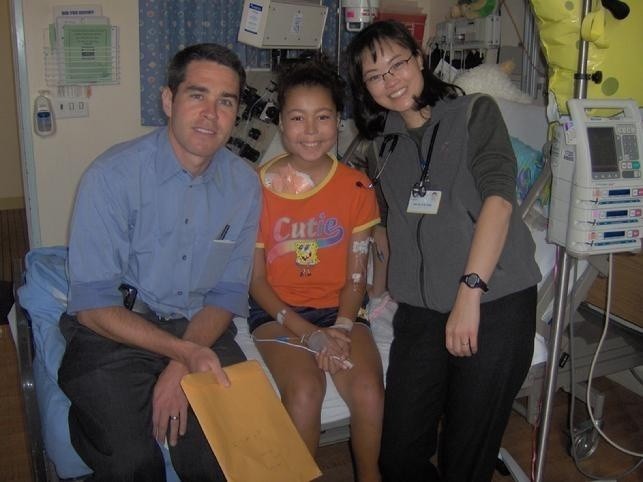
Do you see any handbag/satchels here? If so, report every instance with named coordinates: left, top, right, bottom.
left=57, top=282, right=138, bottom=368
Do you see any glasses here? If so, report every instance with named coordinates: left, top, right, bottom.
left=362, top=50, right=414, bottom=87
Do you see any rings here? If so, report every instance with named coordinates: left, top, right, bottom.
left=460, top=342, right=470, bottom=347
left=168, top=414, right=179, bottom=421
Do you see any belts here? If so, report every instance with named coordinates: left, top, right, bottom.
left=152, top=308, right=186, bottom=322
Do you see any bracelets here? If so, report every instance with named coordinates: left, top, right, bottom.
left=335, top=317, right=354, bottom=334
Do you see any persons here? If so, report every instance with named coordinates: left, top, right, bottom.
left=55, top=41, right=264, bottom=482
left=246, top=50, right=389, bottom=481
left=342, top=18, right=542, bottom=481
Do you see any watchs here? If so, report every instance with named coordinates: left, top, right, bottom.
left=458, top=271, right=489, bottom=294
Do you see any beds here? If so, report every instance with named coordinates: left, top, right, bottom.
left=11, top=101, right=642, bottom=482
